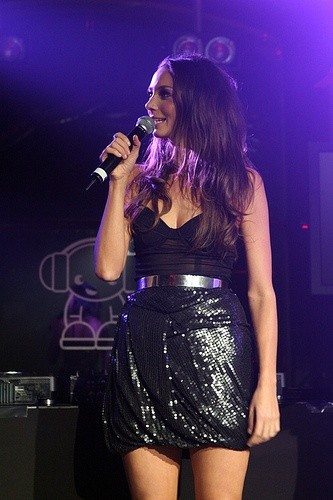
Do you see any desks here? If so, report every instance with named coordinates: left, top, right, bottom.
left=0, top=398, right=333, bottom=499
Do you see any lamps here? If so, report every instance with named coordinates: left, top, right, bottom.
left=149, top=29, right=251, bottom=82
left=44, top=16, right=91, bottom=70
left=1, top=28, right=28, bottom=62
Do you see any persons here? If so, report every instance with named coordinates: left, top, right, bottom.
left=95, top=51, right=282, bottom=500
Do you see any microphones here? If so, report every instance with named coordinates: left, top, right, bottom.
left=85, top=115, right=155, bottom=192
